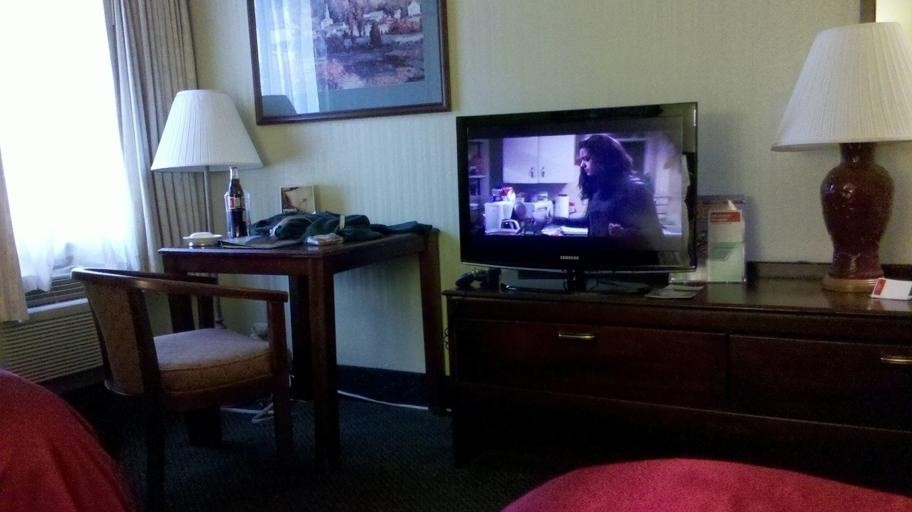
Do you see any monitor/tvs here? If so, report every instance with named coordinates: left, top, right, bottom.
left=456, top=102, right=697, bottom=294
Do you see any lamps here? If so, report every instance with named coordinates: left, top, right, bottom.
left=150, top=89, right=264, bottom=236
left=769, top=21, right=912, bottom=293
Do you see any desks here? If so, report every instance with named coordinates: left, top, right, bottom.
left=157, top=223, right=448, bottom=454
left=437, top=263, right=912, bottom=502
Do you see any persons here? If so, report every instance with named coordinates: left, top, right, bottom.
left=554, top=134, right=665, bottom=237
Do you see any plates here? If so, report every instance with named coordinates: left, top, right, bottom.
left=182, top=235, right=223, bottom=245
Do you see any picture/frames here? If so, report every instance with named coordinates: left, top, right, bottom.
left=246, top=0, right=450, bottom=126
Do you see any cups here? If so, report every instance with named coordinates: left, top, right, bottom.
left=224, top=190, right=251, bottom=236
left=500, top=201, right=514, bottom=219
left=485, top=203, right=502, bottom=231
left=555, top=196, right=569, bottom=218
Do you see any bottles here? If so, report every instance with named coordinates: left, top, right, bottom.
left=228, top=166, right=248, bottom=238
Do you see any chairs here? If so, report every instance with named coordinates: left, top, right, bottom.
left=68, top=266, right=294, bottom=488
